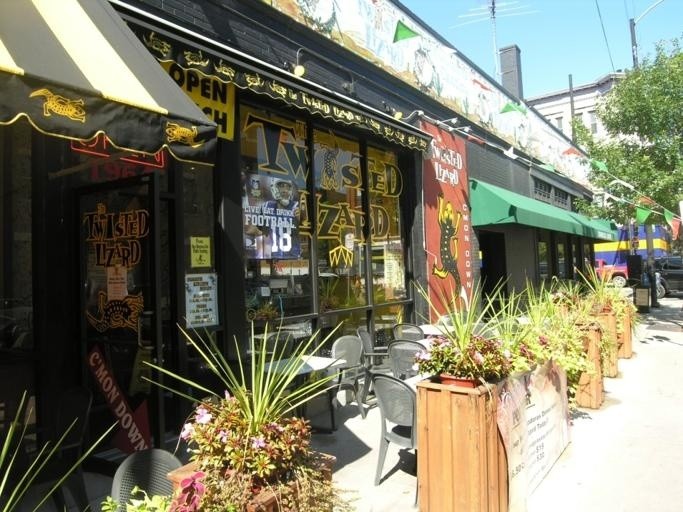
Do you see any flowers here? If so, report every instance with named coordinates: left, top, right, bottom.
left=410, top=272, right=643, bottom=424
left=138, top=322, right=349, bottom=512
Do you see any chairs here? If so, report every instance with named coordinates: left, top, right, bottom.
left=387, top=339, right=426, bottom=381
left=331, top=335, right=366, bottom=418
left=356, top=328, right=390, bottom=403
left=371, top=372, right=417, bottom=508
left=392, top=323, right=424, bottom=341
left=110, top=446, right=184, bottom=512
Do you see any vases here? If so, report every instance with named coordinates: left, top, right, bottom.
left=164, top=453, right=337, bottom=512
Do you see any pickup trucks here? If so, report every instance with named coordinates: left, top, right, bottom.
left=593, top=258, right=629, bottom=288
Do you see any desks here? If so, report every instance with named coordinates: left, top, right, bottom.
left=256, top=355, right=348, bottom=435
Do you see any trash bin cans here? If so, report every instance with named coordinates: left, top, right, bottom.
left=633, top=283, right=652, bottom=313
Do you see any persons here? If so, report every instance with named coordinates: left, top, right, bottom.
left=243, top=177, right=302, bottom=260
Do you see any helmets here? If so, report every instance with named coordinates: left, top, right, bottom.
left=270, top=177, right=294, bottom=206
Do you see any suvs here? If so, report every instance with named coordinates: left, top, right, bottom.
left=645, top=255, right=683, bottom=299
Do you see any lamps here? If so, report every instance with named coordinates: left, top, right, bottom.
left=283, top=48, right=306, bottom=77
left=440, top=116, right=458, bottom=125
left=448, top=126, right=471, bottom=133
left=385, top=102, right=402, bottom=119
